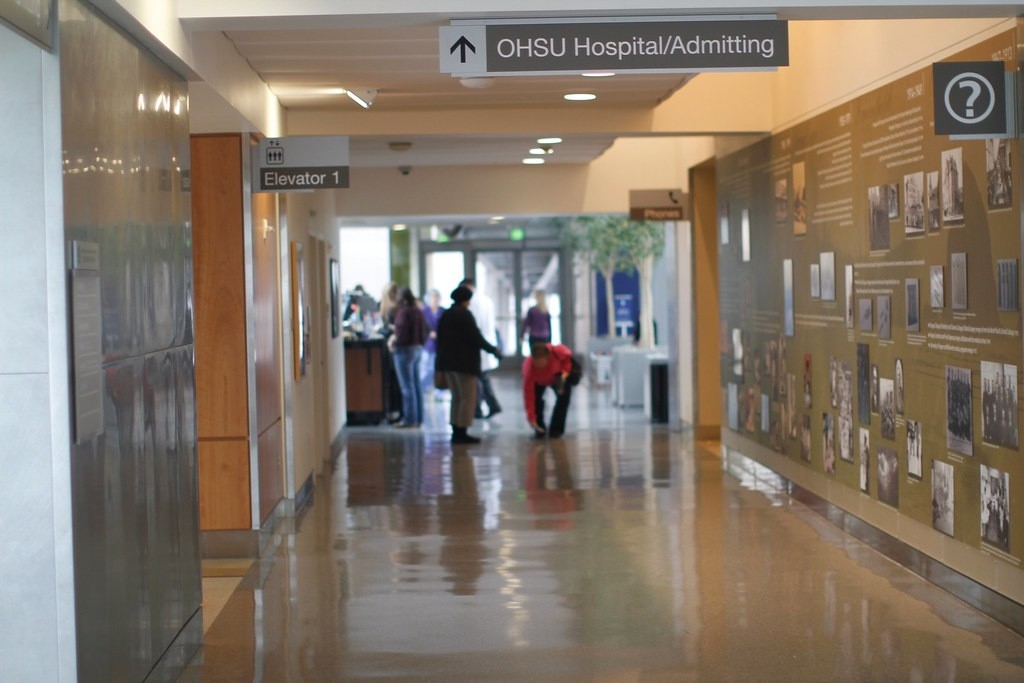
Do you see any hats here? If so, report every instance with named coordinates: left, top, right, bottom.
left=451, top=286, right=473, bottom=300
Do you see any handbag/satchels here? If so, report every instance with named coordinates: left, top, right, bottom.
left=557, top=356, right=581, bottom=384
left=433, top=367, right=449, bottom=388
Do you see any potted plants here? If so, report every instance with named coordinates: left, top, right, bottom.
left=551, top=216, right=669, bottom=408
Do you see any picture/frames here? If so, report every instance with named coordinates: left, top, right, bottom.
left=329, top=258, right=341, bottom=338
left=291, top=239, right=311, bottom=384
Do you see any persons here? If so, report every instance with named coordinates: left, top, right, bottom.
left=881, top=391, right=895, bottom=435
left=981, top=371, right=1018, bottom=446
left=877, top=452, right=895, bottom=492
left=528, top=442, right=576, bottom=534
left=436, top=442, right=489, bottom=597
left=346, top=283, right=448, bottom=428
left=519, top=289, right=552, bottom=347
left=894, top=360, right=903, bottom=412
left=437, top=278, right=506, bottom=444
left=521, top=342, right=574, bottom=437
left=980, top=478, right=1011, bottom=546
left=872, top=368, right=878, bottom=413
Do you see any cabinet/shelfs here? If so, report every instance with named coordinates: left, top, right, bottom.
left=345, top=337, right=389, bottom=426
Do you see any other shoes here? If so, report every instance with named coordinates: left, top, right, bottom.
left=548, top=425, right=563, bottom=437
left=392, top=421, right=421, bottom=428
left=451, top=433, right=480, bottom=444
left=535, top=423, right=546, bottom=438
left=485, top=406, right=501, bottom=418
